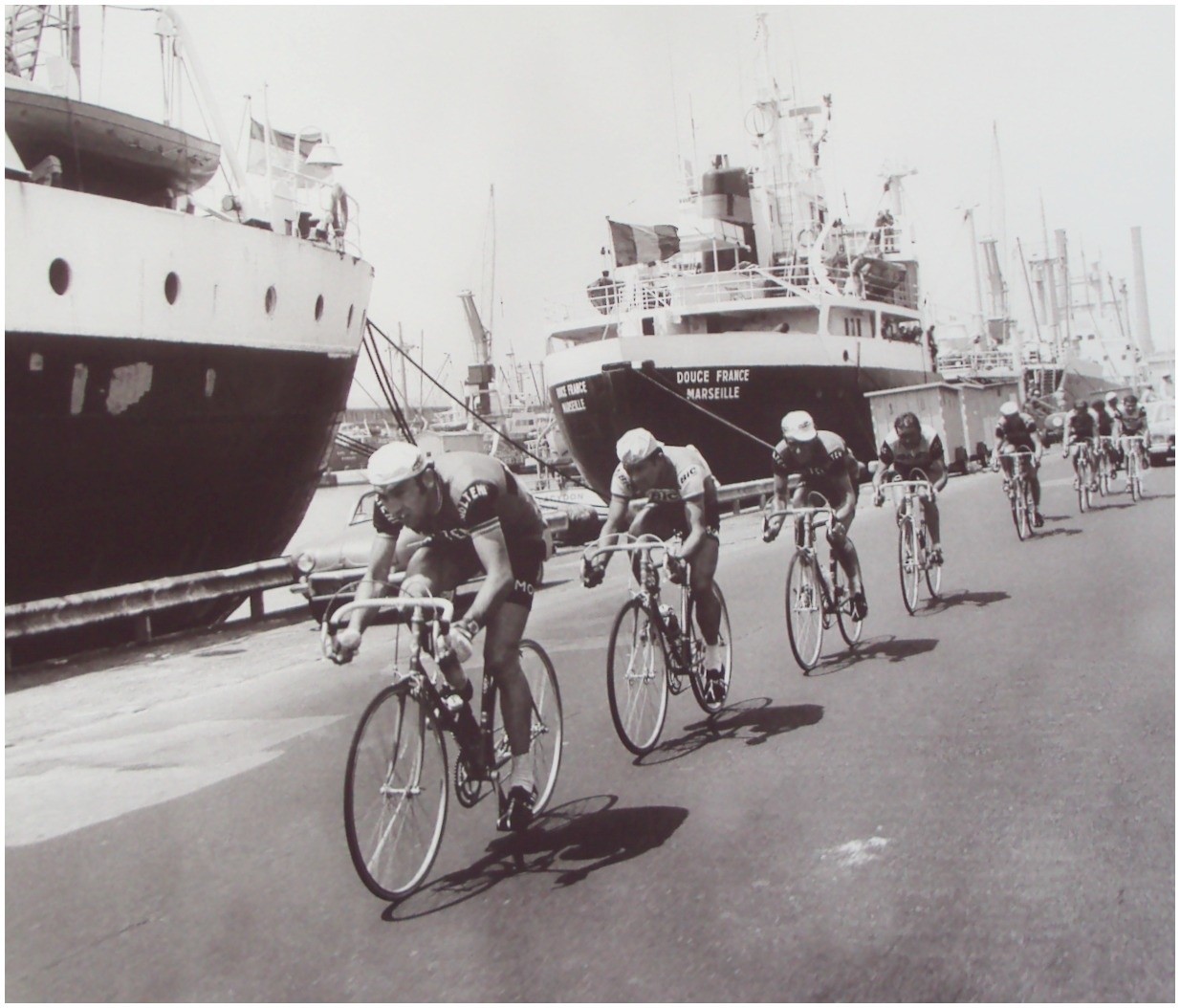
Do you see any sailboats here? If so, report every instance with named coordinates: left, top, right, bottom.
left=316, top=317, right=577, bottom=478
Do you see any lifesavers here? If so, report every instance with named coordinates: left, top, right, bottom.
left=332, top=183, right=348, bottom=238
left=797, top=228, right=818, bottom=247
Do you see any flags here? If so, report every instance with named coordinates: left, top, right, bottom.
left=246, top=116, right=331, bottom=188
left=607, top=219, right=680, bottom=268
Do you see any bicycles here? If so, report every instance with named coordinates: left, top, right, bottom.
left=321, top=569, right=565, bottom=899
left=1067, top=435, right=1145, bottom=513
left=992, top=450, right=1043, bottom=540
left=874, top=468, right=943, bottom=615
left=764, top=504, right=867, bottom=672
left=581, top=527, right=733, bottom=758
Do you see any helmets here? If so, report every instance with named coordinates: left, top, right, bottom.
left=1093, top=398, right=1105, bottom=411
left=1106, top=391, right=1119, bottom=401
left=1074, top=397, right=1087, bottom=410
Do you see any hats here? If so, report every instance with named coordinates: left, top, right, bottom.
left=617, top=428, right=664, bottom=465
left=780, top=411, right=818, bottom=444
left=1000, top=401, right=1019, bottom=417
left=366, top=438, right=430, bottom=488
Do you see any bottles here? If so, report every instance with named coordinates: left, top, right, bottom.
left=439, top=648, right=474, bottom=701
left=657, top=604, right=682, bottom=638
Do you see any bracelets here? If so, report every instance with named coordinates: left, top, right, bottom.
left=453, top=613, right=481, bottom=640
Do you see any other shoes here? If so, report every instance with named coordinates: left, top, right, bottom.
left=1034, top=511, right=1045, bottom=528
left=928, top=551, right=942, bottom=565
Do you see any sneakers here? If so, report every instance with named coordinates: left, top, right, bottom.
left=639, top=612, right=681, bottom=642
left=702, top=664, right=726, bottom=704
left=799, top=586, right=811, bottom=609
left=495, top=781, right=538, bottom=830
left=425, top=680, right=474, bottom=730
left=850, top=589, right=866, bottom=621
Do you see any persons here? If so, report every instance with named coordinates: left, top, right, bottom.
left=990, top=400, right=1044, bottom=527
left=1063, top=399, right=1102, bottom=492
left=872, top=209, right=894, bottom=252
left=872, top=412, right=950, bottom=573
left=581, top=428, right=726, bottom=711
left=1091, top=391, right=1150, bottom=493
left=970, top=334, right=1142, bottom=386
left=851, top=253, right=866, bottom=300
left=882, top=319, right=935, bottom=347
left=324, top=441, right=557, bottom=831
left=760, top=411, right=868, bottom=623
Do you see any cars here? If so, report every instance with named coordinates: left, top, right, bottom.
left=1145, top=401, right=1175, bottom=467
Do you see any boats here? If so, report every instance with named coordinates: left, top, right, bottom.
left=539, top=12, right=932, bottom=503
left=4, top=4, right=373, bottom=697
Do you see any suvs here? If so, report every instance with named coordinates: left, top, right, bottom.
left=1034, top=389, right=1082, bottom=448
